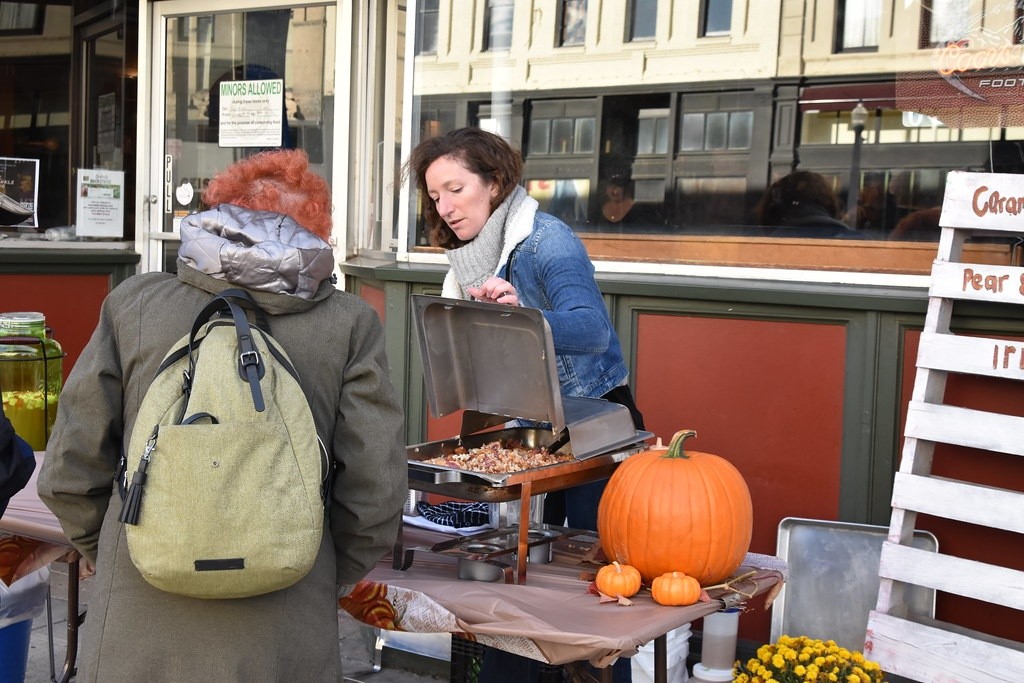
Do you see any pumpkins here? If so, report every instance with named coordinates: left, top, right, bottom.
left=651, top=572, right=700, bottom=606
left=597, top=450, right=752, bottom=585
left=596, top=565, right=641, bottom=597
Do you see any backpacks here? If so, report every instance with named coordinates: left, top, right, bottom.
left=114, top=285, right=338, bottom=601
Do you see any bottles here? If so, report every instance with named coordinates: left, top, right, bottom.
left=489, top=494, right=544, bottom=527
left=686, top=663, right=737, bottom=683
left=1, top=311, right=62, bottom=450
left=702, top=609, right=740, bottom=669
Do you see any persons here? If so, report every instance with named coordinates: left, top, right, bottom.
left=603, top=170, right=945, bottom=244
left=36, top=148, right=409, bottom=683
left=405, top=126, right=647, bottom=531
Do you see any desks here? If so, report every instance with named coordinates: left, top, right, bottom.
left=0, top=445, right=784, bottom=682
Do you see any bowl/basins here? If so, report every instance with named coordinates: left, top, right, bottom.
left=511, top=531, right=553, bottom=564
left=459, top=543, right=504, bottom=581
